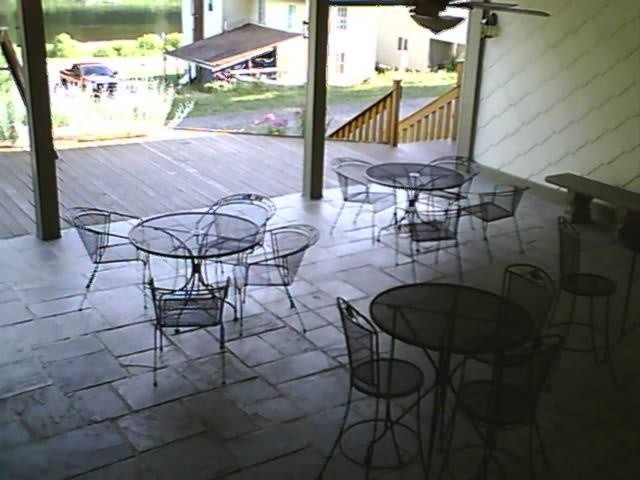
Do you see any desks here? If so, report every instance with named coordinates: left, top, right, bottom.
left=370, top=282, right=533, bottom=480
left=125, top=209, right=265, bottom=333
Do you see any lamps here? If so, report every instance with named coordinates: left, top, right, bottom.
left=409, top=3, right=465, bottom=34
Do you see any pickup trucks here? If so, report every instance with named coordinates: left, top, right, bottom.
left=60, top=63, right=120, bottom=97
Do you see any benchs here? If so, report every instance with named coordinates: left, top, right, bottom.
left=544, top=172, right=640, bottom=225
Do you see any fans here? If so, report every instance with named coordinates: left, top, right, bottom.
left=328, top=0, right=554, bottom=35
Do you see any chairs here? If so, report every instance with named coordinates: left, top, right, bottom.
left=191, top=191, right=278, bottom=324
left=145, top=275, right=233, bottom=388
left=442, top=342, right=565, bottom=480
left=330, top=153, right=526, bottom=283
left=500, top=262, right=554, bottom=371
left=554, top=213, right=618, bottom=360
left=316, top=297, right=430, bottom=479
left=60, top=207, right=156, bottom=312
left=231, top=221, right=320, bottom=337
